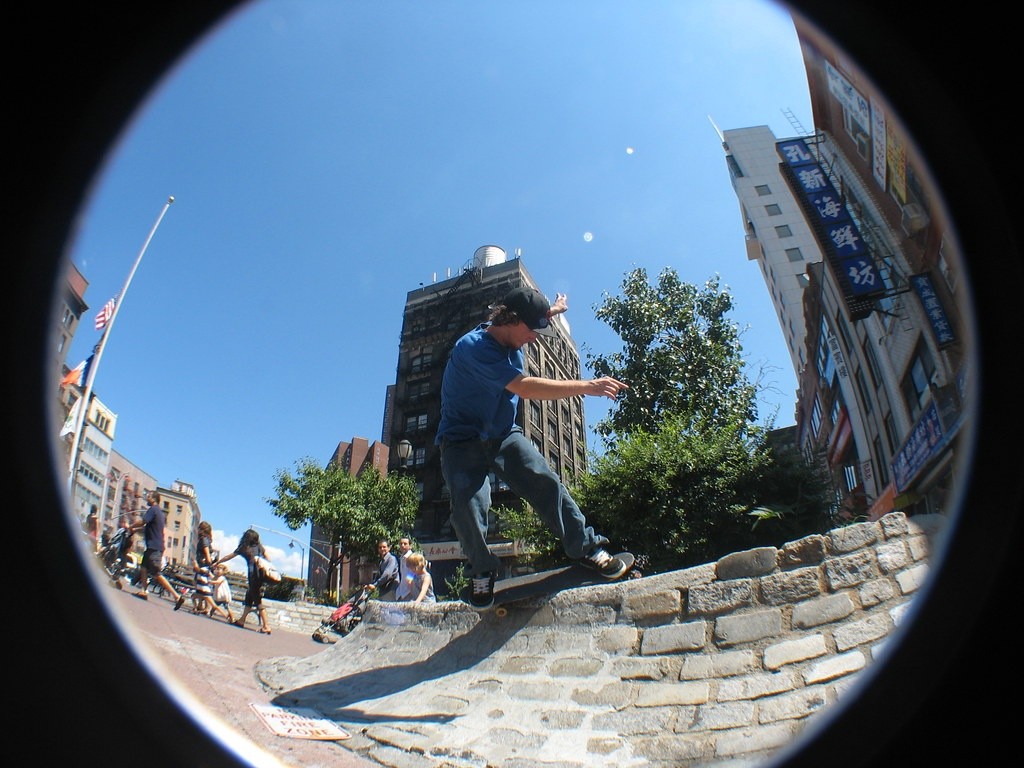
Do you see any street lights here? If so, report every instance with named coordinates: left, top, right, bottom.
left=106, top=468, right=133, bottom=521
left=289, top=539, right=304, bottom=579
left=394, top=439, right=413, bottom=486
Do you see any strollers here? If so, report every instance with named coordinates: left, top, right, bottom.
left=312, top=583, right=376, bottom=644
left=93, top=527, right=135, bottom=590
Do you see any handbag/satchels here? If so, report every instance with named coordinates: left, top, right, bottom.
left=254, top=556, right=281, bottom=582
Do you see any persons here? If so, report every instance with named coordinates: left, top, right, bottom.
left=125, top=490, right=185, bottom=611
left=209, top=529, right=272, bottom=634
left=85, top=504, right=235, bottom=625
left=435, top=288, right=628, bottom=611
left=322, top=535, right=437, bottom=635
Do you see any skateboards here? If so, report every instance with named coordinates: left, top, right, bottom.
left=460, top=550, right=635, bottom=617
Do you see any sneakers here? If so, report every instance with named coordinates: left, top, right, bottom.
left=577, top=547, right=626, bottom=579
left=467, top=568, right=494, bottom=611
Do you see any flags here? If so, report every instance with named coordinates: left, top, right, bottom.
left=95, top=295, right=117, bottom=329
left=60, top=355, right=95, bottom=390
left=58, top=395, right=83, bottom=442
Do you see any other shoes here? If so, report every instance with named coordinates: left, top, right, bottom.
left=131, top=592, right=147, bottom=600
left=174, top=596, right=185, bottom=611
left=225, top=616, right=244, bottom=627
left=256, top=628, right=271, bottom=634
left=190, top=606, right=214, bottom=617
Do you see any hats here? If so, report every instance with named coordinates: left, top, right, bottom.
left=503, top=287, right=558, bottom=338
